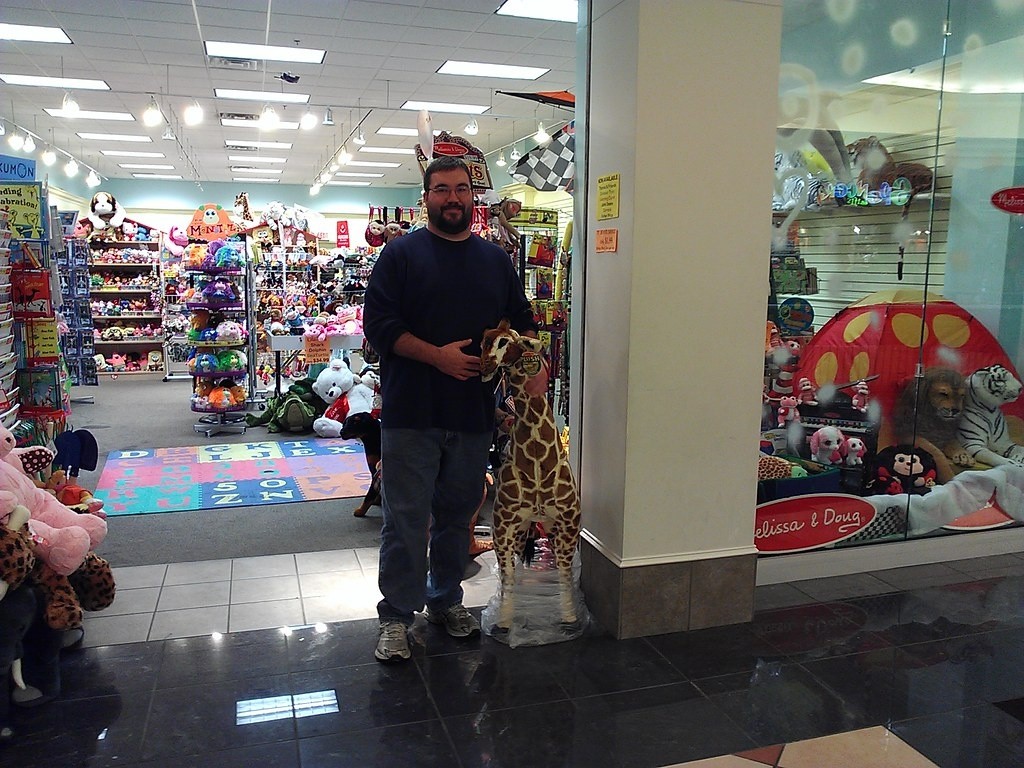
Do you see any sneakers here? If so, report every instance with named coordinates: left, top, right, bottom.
left=375, top=622, right=412, bottom=662
left=424, top=602, right=480, bottom=637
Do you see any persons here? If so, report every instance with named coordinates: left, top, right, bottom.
left=361, top=155, right=542, bottom=661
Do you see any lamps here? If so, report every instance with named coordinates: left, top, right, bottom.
left=0, top=55, right=554, bottom=195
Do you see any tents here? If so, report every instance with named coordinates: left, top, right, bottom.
left=774, top=288, right=1024, bottom=461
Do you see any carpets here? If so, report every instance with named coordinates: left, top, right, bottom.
left=92, top=437, right=374, bottom=517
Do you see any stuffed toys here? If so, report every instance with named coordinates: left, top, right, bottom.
left=479, top=194, right=533, bottom=265
left=478, top=319, right=586, bottom=637
left=0, top=412, right=119, bottom=739
left=72, top=194, right=163, bottom=385
left=171, top=193, right=433, bottom=543
left=758, top=133, right=1024, bottom=520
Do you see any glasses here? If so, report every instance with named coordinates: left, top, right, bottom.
left=428, top=185, right=472, bottom=196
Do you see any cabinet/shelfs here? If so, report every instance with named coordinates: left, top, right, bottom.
left=10, top=238, right=99, bottom=435
left=85, top=233, right=167, bottom=375
left=161, top=231, right=260, bottom=382
left=238, top=218, right=381, bottom=398
left=512, top=224, right=558, bottom=303
left=776, top=192, right=951, bottom=302
left=188, top=234, right=251, bottom=439
